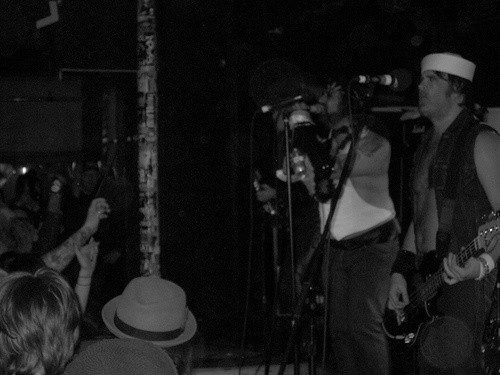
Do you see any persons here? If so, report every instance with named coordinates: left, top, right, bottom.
left=0, top=161, right=196, bottom=375
left=388, top=52, right=500, bottom=375
left=256, top=105, right=322, bottom=301
left=288, top=72, right=399, bottom=375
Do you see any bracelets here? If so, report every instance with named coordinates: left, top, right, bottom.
left=77, top=275, right=92, bottom=286
left=478, top=256, right=491, bottom=273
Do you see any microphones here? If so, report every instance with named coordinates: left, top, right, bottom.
left=354, top=67, right=412, bottom=91
left=274, top=87, right=319, bottom=108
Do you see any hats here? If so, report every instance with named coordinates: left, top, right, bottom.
left=421, top=52, right=476, bottom=82
left=83, top=161, right=97, bottom=170
left=102, top=277, right=198, bottom=347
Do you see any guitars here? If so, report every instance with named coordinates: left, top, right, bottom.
left=382, top=210, right=500, bottom=340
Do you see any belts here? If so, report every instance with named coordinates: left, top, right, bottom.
left=327, top=220, right=392, bottom=250
